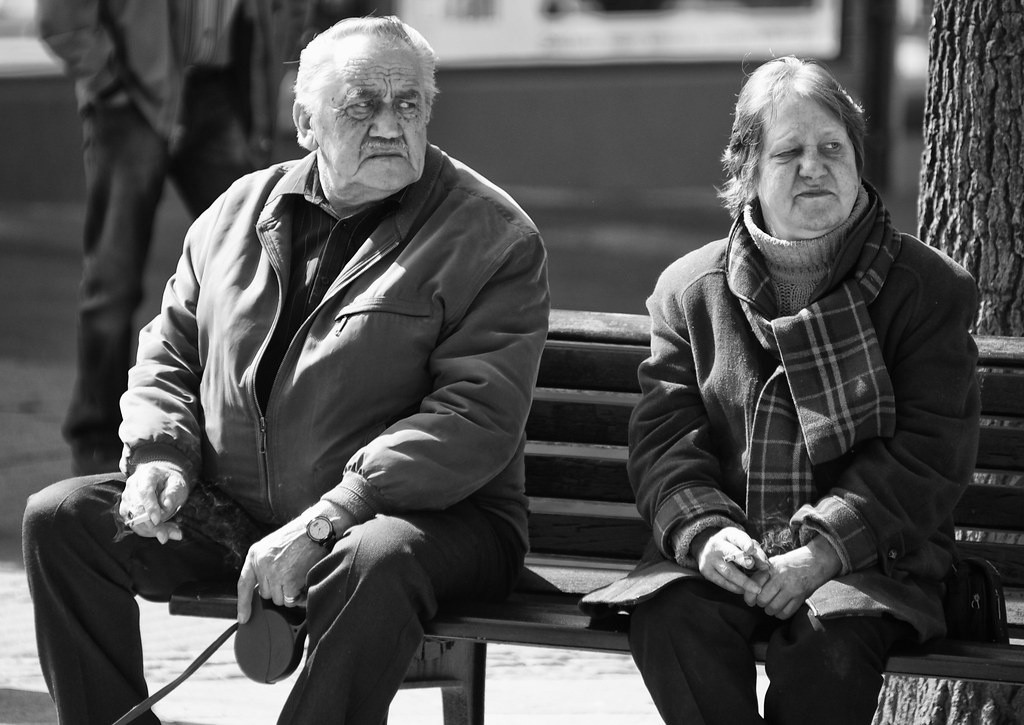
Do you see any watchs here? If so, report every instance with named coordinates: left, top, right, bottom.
left=301, top=505, right=336, bottom=549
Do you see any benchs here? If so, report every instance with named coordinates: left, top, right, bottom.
left=168, top=307, right=1024, bottom=725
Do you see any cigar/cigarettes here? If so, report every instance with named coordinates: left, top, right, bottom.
left=725, top=549, right=757, bottom=563
left=126, top=507, right=165, bottom=527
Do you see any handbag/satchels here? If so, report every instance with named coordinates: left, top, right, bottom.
left=942, top=547, right=1010, bottom=647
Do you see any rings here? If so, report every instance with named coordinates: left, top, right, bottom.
left=283, top=591, right=303, bottom=604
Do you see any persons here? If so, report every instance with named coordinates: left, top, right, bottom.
left=580, top=58, right=984, bottom=725
left=32, top=0, right=385, bottom=475
left=24, top=16, right=551, bottom=725
